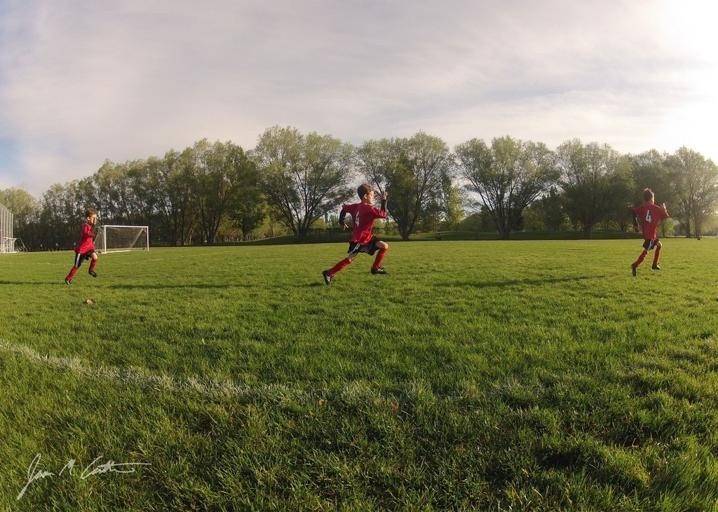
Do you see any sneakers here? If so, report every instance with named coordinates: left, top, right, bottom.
left=322, top=270, right=332, bottom=285
left=371, top=267, right=386, bottom=274
left=65, top=270, right=98, bottom=286
left=631, top=263, right=662, bottom=277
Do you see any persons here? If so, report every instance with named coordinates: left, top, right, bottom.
left=322, top=183, right=389, bottom=284
left=630, top=188, right=670, bottom=277
left=65, top=207, right=100, bottom=286
